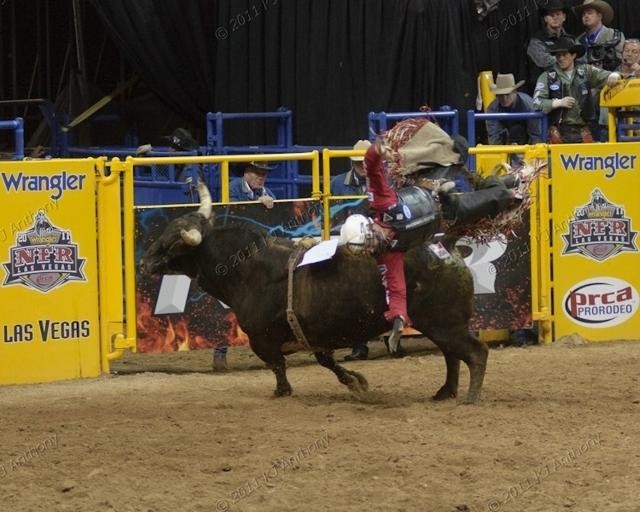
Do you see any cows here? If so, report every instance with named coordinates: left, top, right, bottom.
left=137, top=180, right=490, bottom=407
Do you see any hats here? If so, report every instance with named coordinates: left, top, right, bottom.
left=576, top=0, right=614, bottom=25
left=162, top=126, right=201, bottom=152
left=538, top=1, right=571, bottom=16
left=547, top=36, right=587, bottom=57
left=349, top=138, right=372, bottom=162
left=488, top=72, right=526, bottom=97
left=248, top=158, right=280, bottom=171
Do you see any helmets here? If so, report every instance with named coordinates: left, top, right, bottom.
left=339, top=213, right=383, bottom=260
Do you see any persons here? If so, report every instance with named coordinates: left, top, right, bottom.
left=355, top=133, right=519, bottom=352
left=225, top=153, right=278, bottom=212
left=485, top=71, right=542, bottom=146
left=136, top=128, right=203, bottom=183
left=615, top=39, right=640, bottom=78
left=533, top=37, right=621, bottom=143
left=526, top=0, right=574, bottom=97
left=30, top=145, right=46, bottom=157
left=326, top=138, right=378, bottom=201
left=565, top=0, right=625, bottom=141
left=379, top=119, right=469, bottom=192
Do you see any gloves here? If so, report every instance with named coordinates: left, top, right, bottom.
left=553, top=96, right=575, bottom=109
left=135, top=143, right=156, bottom=155
left=387, top=314, right=406, bottom=355
left=377, top=143, right=400, bottom=163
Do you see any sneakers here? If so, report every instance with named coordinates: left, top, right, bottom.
left=343, top=335, right=407, bottom=360
left=485, top=173, right=524, bottom=190
